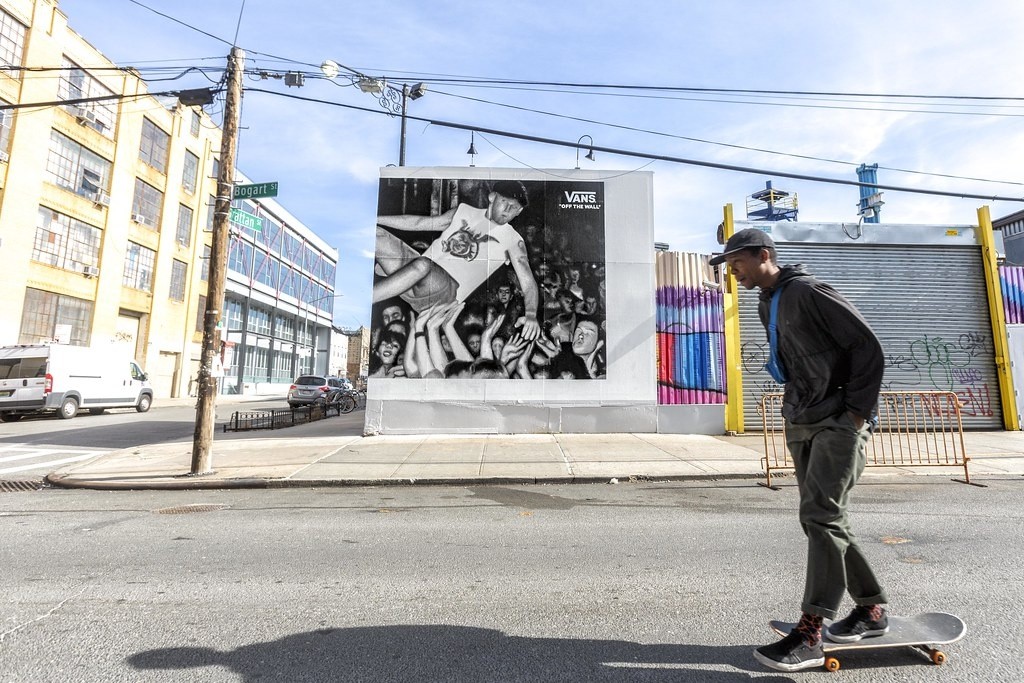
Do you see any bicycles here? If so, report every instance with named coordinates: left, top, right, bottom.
left=311, top=383, right=366, bottom=413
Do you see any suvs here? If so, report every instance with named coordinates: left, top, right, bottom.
left=287, top=375, right=354, bottom=411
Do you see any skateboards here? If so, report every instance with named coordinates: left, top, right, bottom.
left=768, top=611, right=968, bottom=674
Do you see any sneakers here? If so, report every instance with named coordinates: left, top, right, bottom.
left=826, top=605, right=889, bottom=643
left=753, top=629, right=825, bottom=671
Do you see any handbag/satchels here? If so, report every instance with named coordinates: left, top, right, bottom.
left=764, top=285, right=786, bottom=385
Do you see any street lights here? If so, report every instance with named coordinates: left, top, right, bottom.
left=320, top=60, right=426, bottom=167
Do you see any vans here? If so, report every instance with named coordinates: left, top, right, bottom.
left=0, top=344, right=154, bottom=420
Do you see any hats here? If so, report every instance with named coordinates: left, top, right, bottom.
left=709, top=228, right=775, bottom=265
left=492, top=181, right=529, bottom=207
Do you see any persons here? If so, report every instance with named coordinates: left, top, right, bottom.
left=371, top=179, right=607, bottom=381
left=708, top=227, right=890, bottom=671
